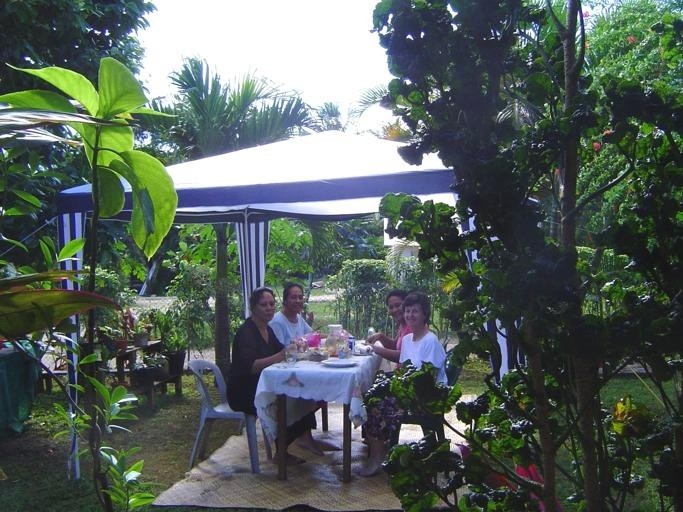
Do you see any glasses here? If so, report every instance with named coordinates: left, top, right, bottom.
left=254, top=286, right=273, bottom=296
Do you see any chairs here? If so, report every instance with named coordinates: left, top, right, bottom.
left=188, top=360, right=273, bottom=474
left=391, top=348, right=462, bottom=478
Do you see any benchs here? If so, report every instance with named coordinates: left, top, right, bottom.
left=41, top=369, right=130, bottom=390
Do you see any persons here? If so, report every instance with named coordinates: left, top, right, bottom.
left=270, top=284, right=321, bottom=355
left=351, top=290, right=450, bottom=477
left=228, top=285, right=316, bottom=467
left=364, top=292, right=405, bottom=371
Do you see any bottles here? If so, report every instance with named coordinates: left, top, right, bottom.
left=367, top=326, right=376, bottom=344
left=348, top=336, right=355, bottom=351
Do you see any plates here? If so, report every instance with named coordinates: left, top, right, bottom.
left=320, top=357, right=358, bottom=367
left=353, top=351, right=369, bottom=356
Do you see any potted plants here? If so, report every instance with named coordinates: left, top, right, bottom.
left=112, top=287, right=193, bottom=389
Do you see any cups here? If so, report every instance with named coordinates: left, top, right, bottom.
left=284, top=347, right=297, bottom=368
left=359, top=346, right=370, bottom=354
